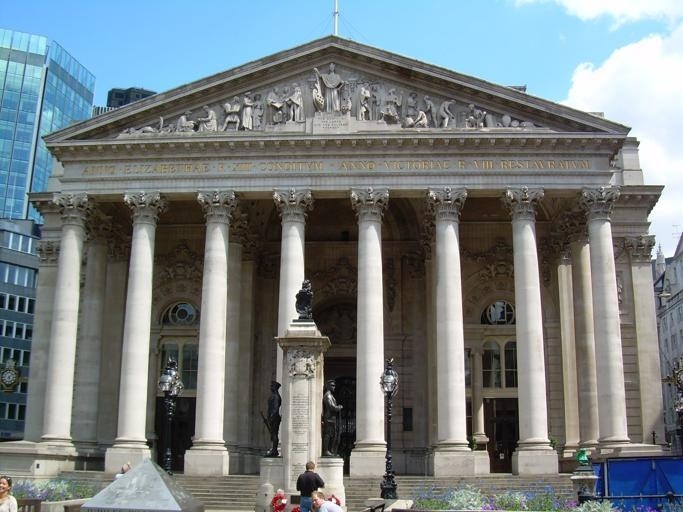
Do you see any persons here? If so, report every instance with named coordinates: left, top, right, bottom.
left=295, top=279, right=315, bottom=319
left=322, top=379, right=343, bottom=456
left=123, top=63, right=485, bottom=133
left=296, top=462, right=324, bottom=512
left=311, top=490, right=343, bottom=511
left=0, top=476, right=18, bottom=511
left=265, top=381, right=281, bottom=457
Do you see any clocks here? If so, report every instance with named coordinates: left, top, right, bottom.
left=2, top=369, right=17, bottom=386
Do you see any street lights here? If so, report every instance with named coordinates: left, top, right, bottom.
left=379, top=356, right=400, bottom=499
left=158, top=357, right=184, bottom=476
